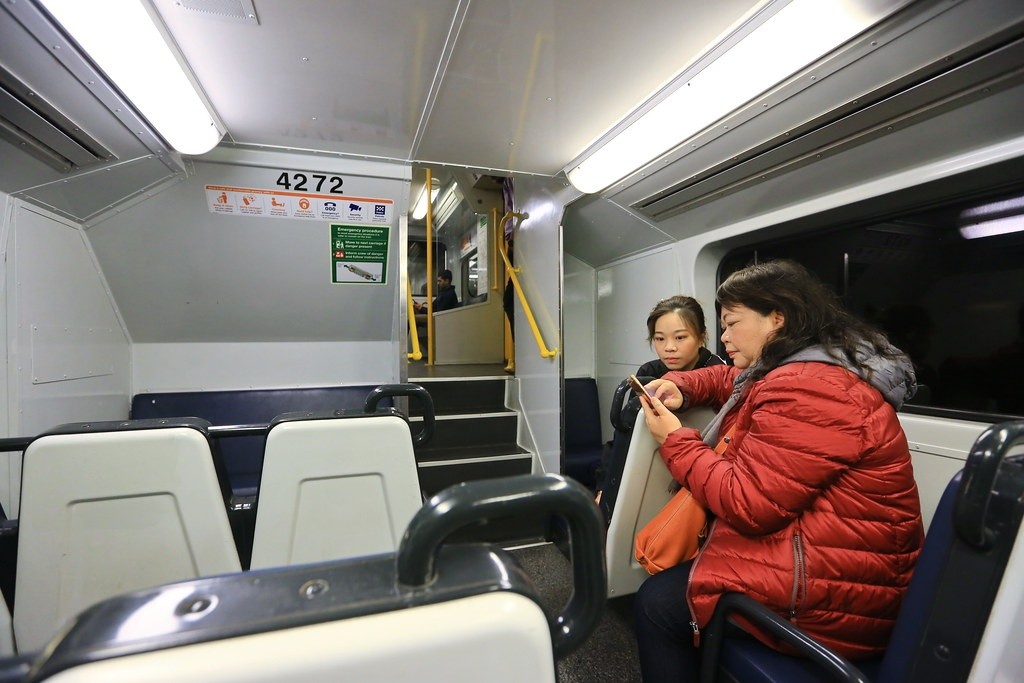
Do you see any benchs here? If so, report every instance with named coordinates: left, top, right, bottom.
left=458, top=293, right=487, bottom=306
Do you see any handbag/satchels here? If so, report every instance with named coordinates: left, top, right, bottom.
left=634, top=486, right=709, bottom=576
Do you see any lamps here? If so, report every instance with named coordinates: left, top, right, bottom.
left=412, top=185, right=441, bottom=220
left=564, top=0, right=914, bottom=196
left=30, top=0, right=230, bottom=155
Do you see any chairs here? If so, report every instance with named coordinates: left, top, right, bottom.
left=0, top=376, right=1023, bottom=683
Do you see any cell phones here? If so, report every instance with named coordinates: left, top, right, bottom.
left=627, top=375, right=655, bottom=409
left=413, top=299, right=417, bottom=304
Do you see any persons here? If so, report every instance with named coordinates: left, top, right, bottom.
left=406, top=270, right=459, bottom=337
left=591, top=295, right=727, bottom=510
left=633, top=258, right=926, bottom=683
